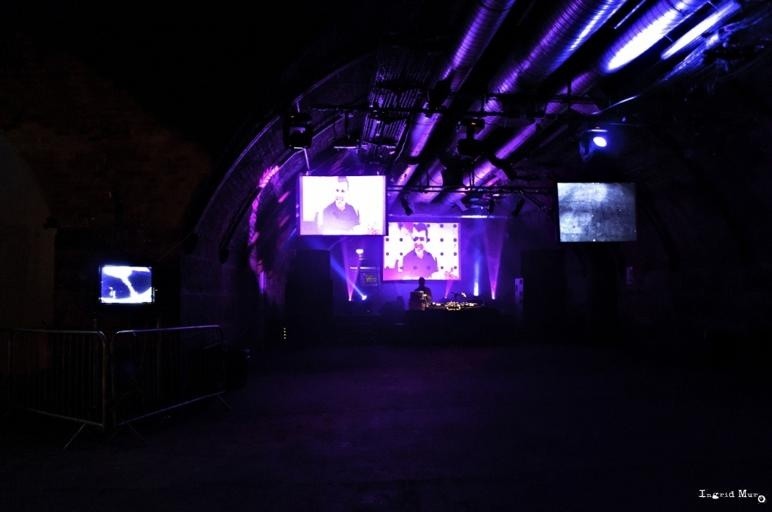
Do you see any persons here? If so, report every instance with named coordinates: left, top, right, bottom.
left=626, top=250, right=659, bottom=353
left=324, top=176, right=359, bottom=225
left=403, top=224, right=438, bottom=277
left=415, top=277, right=431, bottom=296
